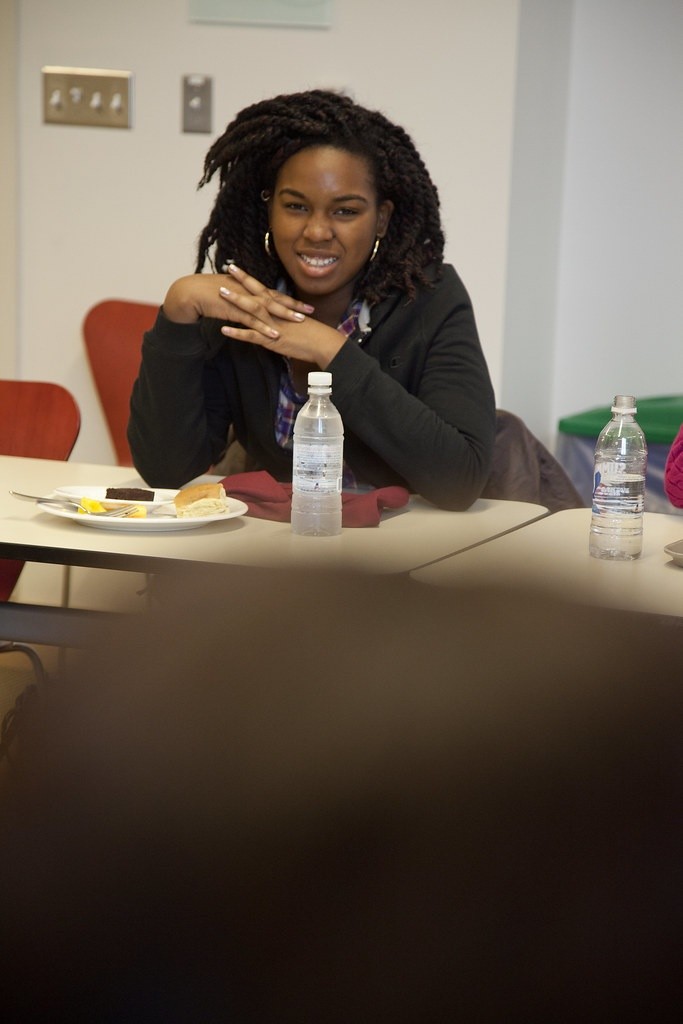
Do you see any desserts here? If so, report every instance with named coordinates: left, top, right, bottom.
left=77, top=498, right=146, bottom=518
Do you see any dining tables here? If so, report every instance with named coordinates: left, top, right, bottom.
left=0, top=453, right=555, bottom=643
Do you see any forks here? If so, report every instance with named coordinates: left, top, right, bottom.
left=9, top=491, right=138, bottom=519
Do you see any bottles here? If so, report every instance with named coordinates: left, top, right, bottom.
left=589, top=396, right=647, bottom=559
left=291, top=372, right=343, bottom=537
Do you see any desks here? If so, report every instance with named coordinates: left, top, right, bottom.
left=408, top=507, right=683, bottom=622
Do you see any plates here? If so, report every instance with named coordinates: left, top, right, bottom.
left=663, top=539, right=683, bottom=567
left=36, top=488, right=247, bottom=532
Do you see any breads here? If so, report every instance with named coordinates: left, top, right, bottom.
left=105, top=487, right=155, bottom=502
left=173, top=482, right=231, bottom=518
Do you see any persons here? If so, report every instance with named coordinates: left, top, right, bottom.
left=663, top=423, right=683, bottom=508
left=125, top=89, right=497, bottom=512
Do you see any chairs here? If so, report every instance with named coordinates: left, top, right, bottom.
left=83, top=300, right=162, bottom=470
left=477, top=409, right=584, bottom=515
left=0, top=379, right=82, bottom=687
